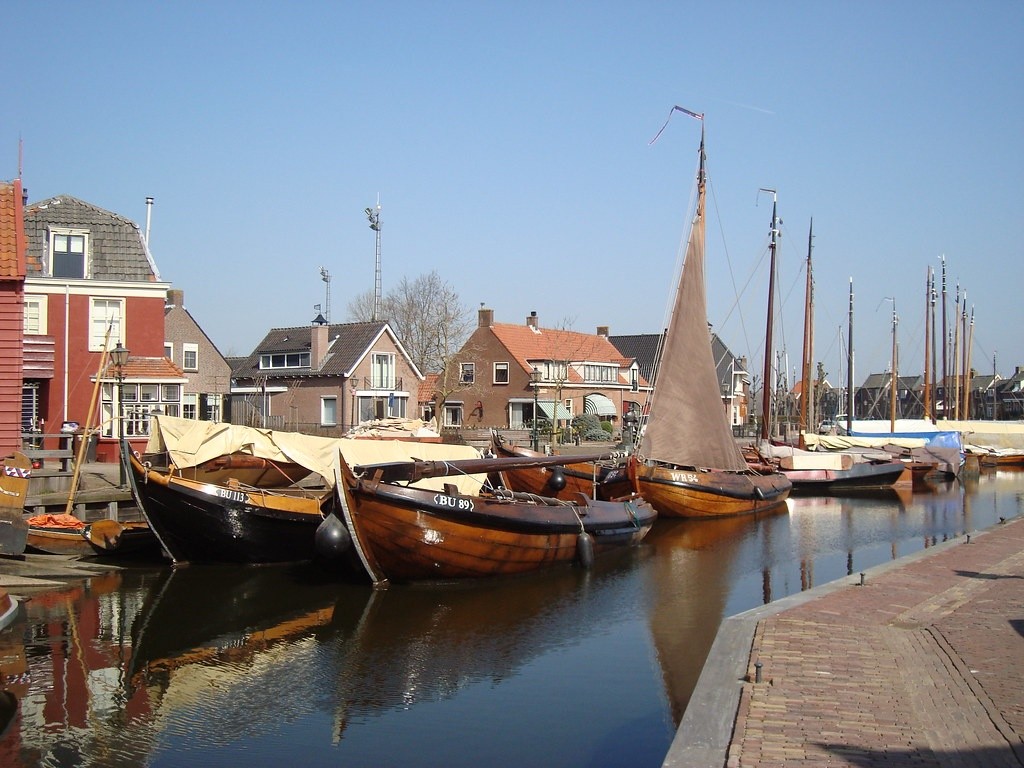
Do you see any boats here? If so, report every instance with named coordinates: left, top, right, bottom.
left=492, top=433, right=626, bottom=505
left=336, top=444, right=658, bottom=592
left=123, top=412, right=499, bottom=572
left=118, top=570, right=335, bottom=702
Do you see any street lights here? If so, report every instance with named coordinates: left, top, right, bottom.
left=108, top=339, right=130, bottom=489
left=530, top=364, right=542, bottom=452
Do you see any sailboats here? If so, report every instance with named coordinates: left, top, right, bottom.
left=743, top=187, right=1024, bottom=488
left=598, top=105, right=793, bottom=520
left=28, top=312, right=149, bottom=557
